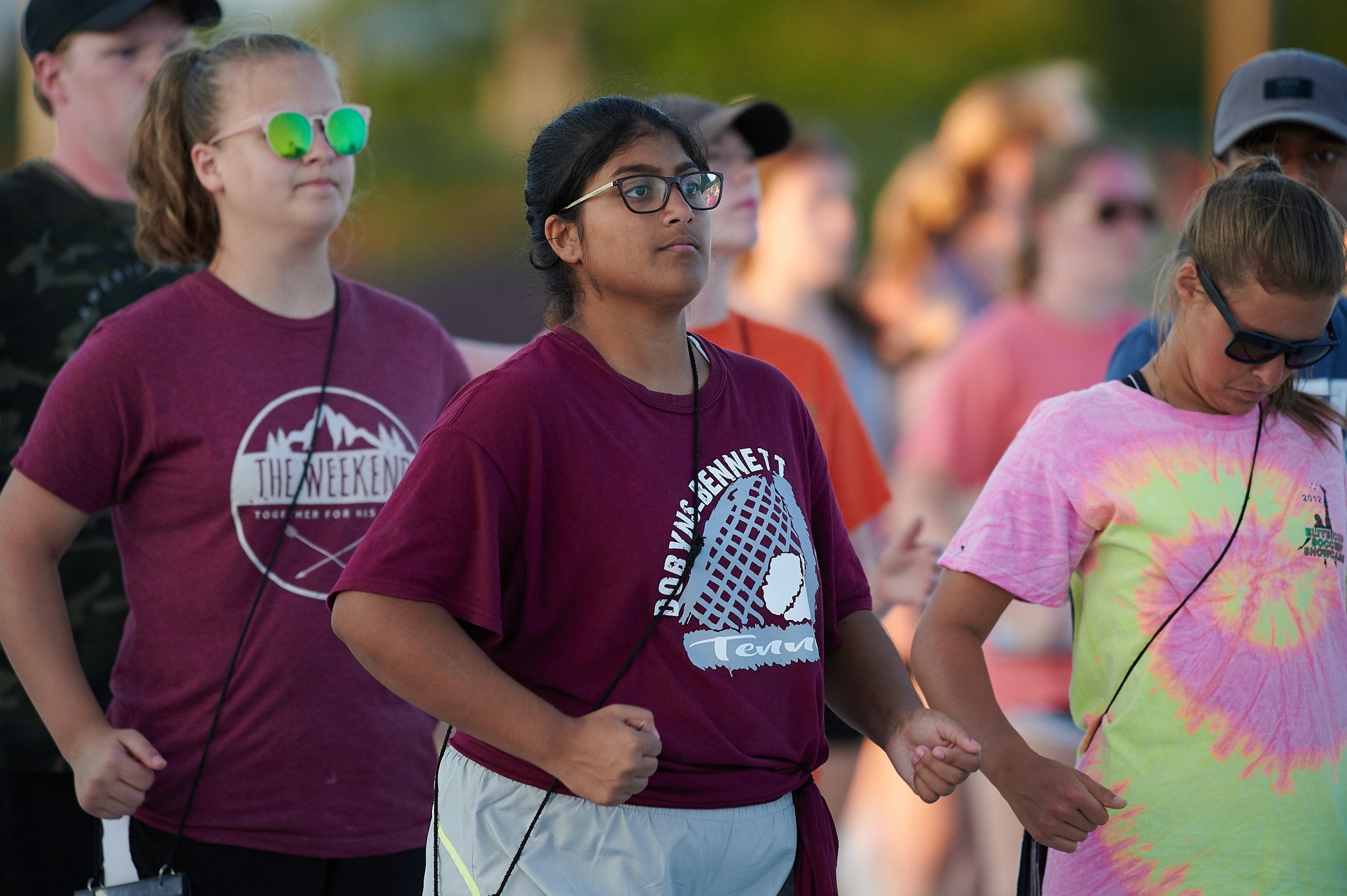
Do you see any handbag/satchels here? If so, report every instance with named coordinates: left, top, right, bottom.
left=73, top=865, right=191, bottom=896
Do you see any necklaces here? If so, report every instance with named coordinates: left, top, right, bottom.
left=1149, top=356, right=1171, bottom=405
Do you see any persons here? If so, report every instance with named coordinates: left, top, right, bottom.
left=0, top=1, right=1347, bottom=896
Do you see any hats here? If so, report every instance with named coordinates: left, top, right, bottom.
left=20, top=0, right=223, bottom=64
left=645, top=97, right=797, bottom=163
left=1212, top=50, right=1347, bottom=158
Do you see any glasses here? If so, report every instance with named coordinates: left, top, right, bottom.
left=559, top=172, right=725, bottom=214
left=1079, top=198, right=1164, bottom=228
left=207, top=104, right=371, bottom=160
left=1179, top=239, right=1342, bottom=369
